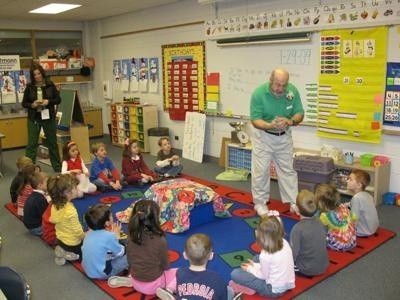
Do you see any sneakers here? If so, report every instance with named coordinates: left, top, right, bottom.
left=253, top=205, right=269, bottom=217
left=54, top=245, right=79, bottom=261
left=290, top=202, right=300, bottom=216
left=229, top=280, right=256, bottom=296
left=107, top=276, right=133, bottom=288
left=155, top=288, right=176, bottom=300
left=54, top=258, right=66, bottom=265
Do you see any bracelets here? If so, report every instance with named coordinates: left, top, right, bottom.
left=292, top=117, right=300, bottom=126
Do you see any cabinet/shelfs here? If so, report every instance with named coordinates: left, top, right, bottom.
left=110, top=103, right=158, bottom=153
left=224, top=140, right=390, bottom=207
left=83, top=107, right=103, bottom=137
left=0, top=115, right=42, bottom=152
left=39, top=123, right=94, bottom=167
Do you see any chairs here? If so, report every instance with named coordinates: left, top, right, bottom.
left=0, top=265, right=32, bottom=300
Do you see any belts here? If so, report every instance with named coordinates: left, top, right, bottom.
left=264, top=130, right=285, bottom=136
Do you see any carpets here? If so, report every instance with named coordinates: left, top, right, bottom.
left=5, top=172, right=396, bottom=300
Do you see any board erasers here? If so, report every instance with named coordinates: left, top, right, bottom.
left=58, top=124, right=69, bottom=131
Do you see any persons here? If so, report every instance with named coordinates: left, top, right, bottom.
left=344, top=170, right=380, bottom=238
left=107, top=199, right=179, bottom=300
left=229, top=214, right=296, bottom=299
left=122, top=138, right=159, bottom=187
left=174, top=233, right=244, bottom=300
left=22, top=64, right=62, bottom=173
left=152, top=136, right=183, bottom=180
left=9, top=155, right=85, bottom=266
left=288, top=190, right=329, bottom=278
left=249, top=67, right=304, bottom=217
left=89, top=143, right=125, bottom=191
left=79, top=203, right=129, bottom=280
left=315, top=183, right=359, bottom=253
left=61, top=141, right=97, bottom=199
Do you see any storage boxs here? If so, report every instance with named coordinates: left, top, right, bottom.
left=298, top=172, right=335, bottom=184
left=293, top=155, right=335, bottom=172
left=297, top=184, right=318, bottom=193
left=67, top=59, right=82, bottom=69
left=32, top=58, right=67, bottom=71
left=382, top=192, right=397, bottom=205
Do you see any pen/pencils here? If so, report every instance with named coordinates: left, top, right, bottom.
left=344, top=152, right=354, bottom=157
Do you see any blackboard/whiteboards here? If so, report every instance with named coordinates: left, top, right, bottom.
left=72, top=89, right=88, bottom=126
left=205, top=23, right=400, bottom=136
left=56, top=89, right=77, bottom=132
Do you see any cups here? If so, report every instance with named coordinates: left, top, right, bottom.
left=344, top=151, right=355, bottom=165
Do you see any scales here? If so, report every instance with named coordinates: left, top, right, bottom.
left=229, top=121, right=249, bottom=147
